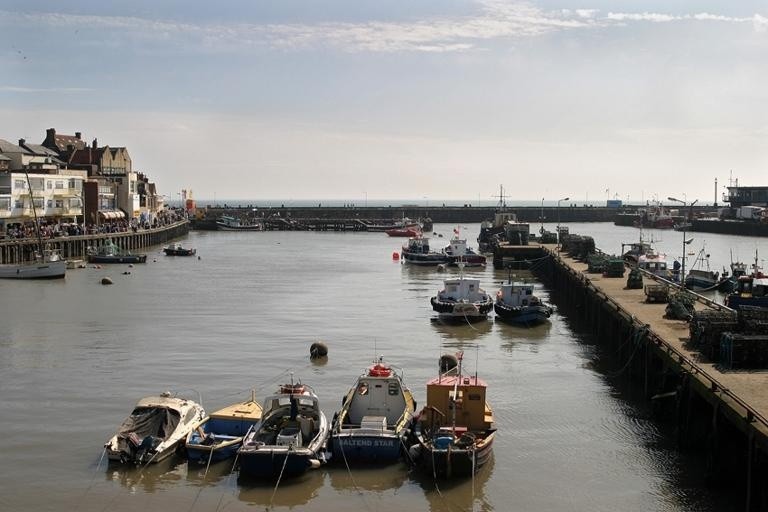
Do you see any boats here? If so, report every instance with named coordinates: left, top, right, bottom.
left=327, top=353, right=416, bottom=463
left=85, top=244, right=146, bottom=264
left=235, top=371, right=330, bottom=476
left=621, top=224, right=767, bottom=309
left=184, top=389, right=262, bottom=468
left=385, top=185, right=553, bottom=328
left=413, top=350, right=495, bottom=483
left=162, top=243, right=195, bottom=256
left=0, top=262, right=67, bottom=279
left=103, top=389, right=208, bottom=466
left=215, top=215, right=260, bottom=231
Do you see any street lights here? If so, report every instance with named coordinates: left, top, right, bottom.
left=667, top=196, right=686, bottom=290
left=557, top=197, right=568, bottom=257
